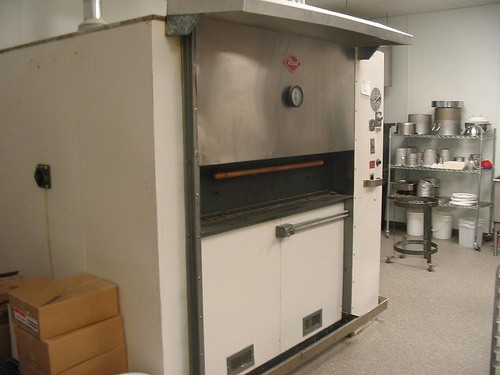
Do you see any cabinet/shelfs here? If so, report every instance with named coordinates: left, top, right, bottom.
left=385, top=126, right=497, bottom=251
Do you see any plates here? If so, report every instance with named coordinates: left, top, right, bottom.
left=448, top=192, right=477, bottom=206
left=469, top=118, right=489, bottom=121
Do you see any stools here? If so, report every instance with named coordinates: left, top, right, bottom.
left=387, top=197, right=439, bottom=272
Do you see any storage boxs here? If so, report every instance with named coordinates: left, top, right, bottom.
left=7, top=274, right=120, bottom=337
left=13, top=315, right=124, bottom=373
left=16, top=346, right=128, bottom=375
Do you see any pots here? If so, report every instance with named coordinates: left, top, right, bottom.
left=432, top=108, right=461, bottom=136
left=394, top=121, right=418, bottom=135
left=396, top=181, right=417, bottom=196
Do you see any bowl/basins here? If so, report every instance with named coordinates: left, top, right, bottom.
left=463, top=125, right=480, bottom=135
left=472, top=114, right=481, bottom=117
left=465, top=123, right=491, bottom=134
